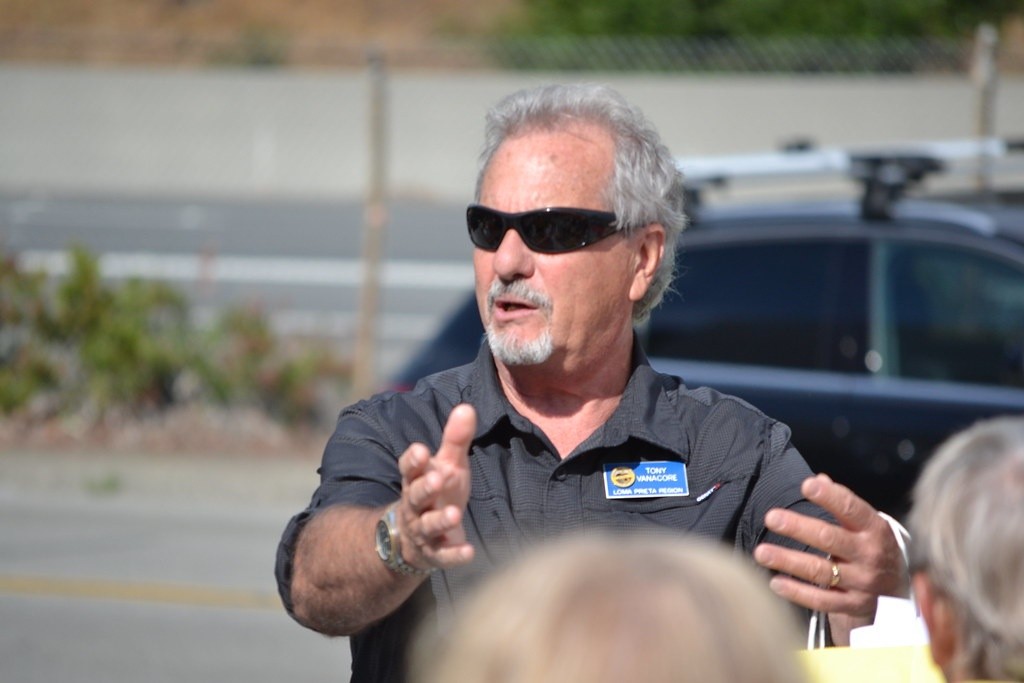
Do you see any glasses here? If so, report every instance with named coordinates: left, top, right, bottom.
left=467, top=203, right=654, bottom=253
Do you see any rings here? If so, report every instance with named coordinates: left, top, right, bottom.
left=827, top=561, right=839, bottom=591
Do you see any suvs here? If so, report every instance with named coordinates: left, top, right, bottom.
left=370, top=134, right=1024, bottom=580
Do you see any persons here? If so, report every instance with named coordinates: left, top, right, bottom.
left=272, top=79, right=1024, bottom=683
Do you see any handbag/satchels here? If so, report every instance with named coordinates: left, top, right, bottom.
left=793, top=512, right=947, bottom=683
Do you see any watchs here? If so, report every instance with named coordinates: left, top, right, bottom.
left=373, top=499, right=438, bottom=580
